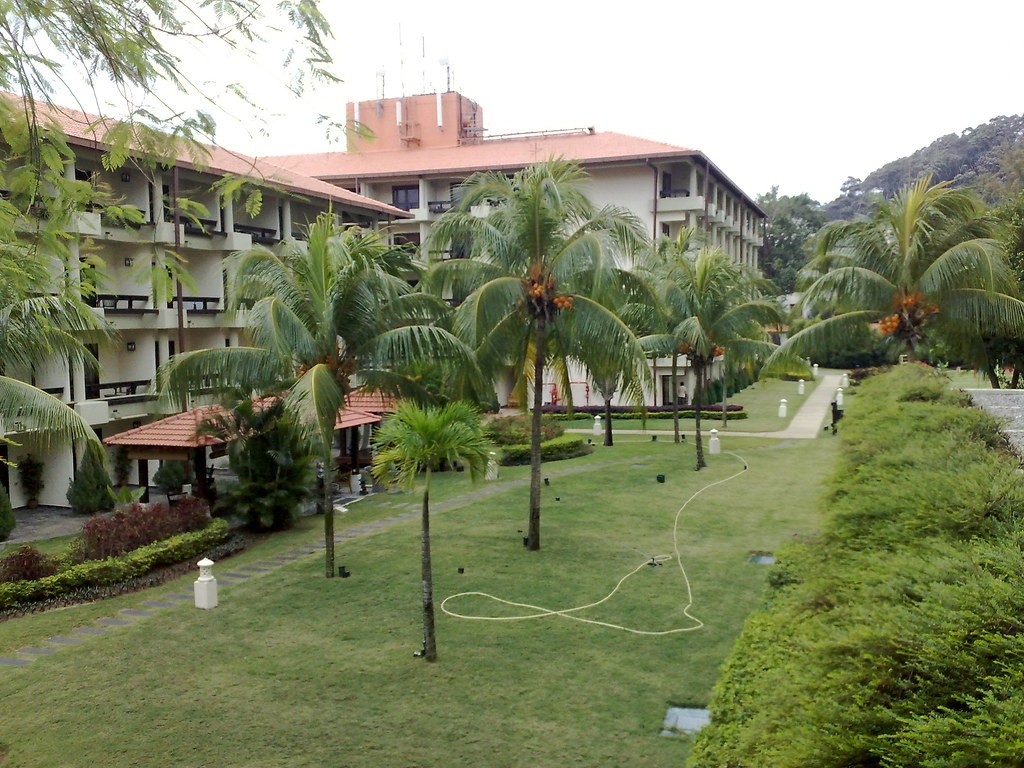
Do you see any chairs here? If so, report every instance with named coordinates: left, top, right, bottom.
left=336, top=468, right=353, bottom=494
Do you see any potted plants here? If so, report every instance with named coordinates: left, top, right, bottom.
left=17, top=453, right=45, bottom=509
left=115, top=447, right=131, bottom=486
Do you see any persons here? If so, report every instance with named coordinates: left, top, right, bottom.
left=678, top=382, right=688, bottom=405
